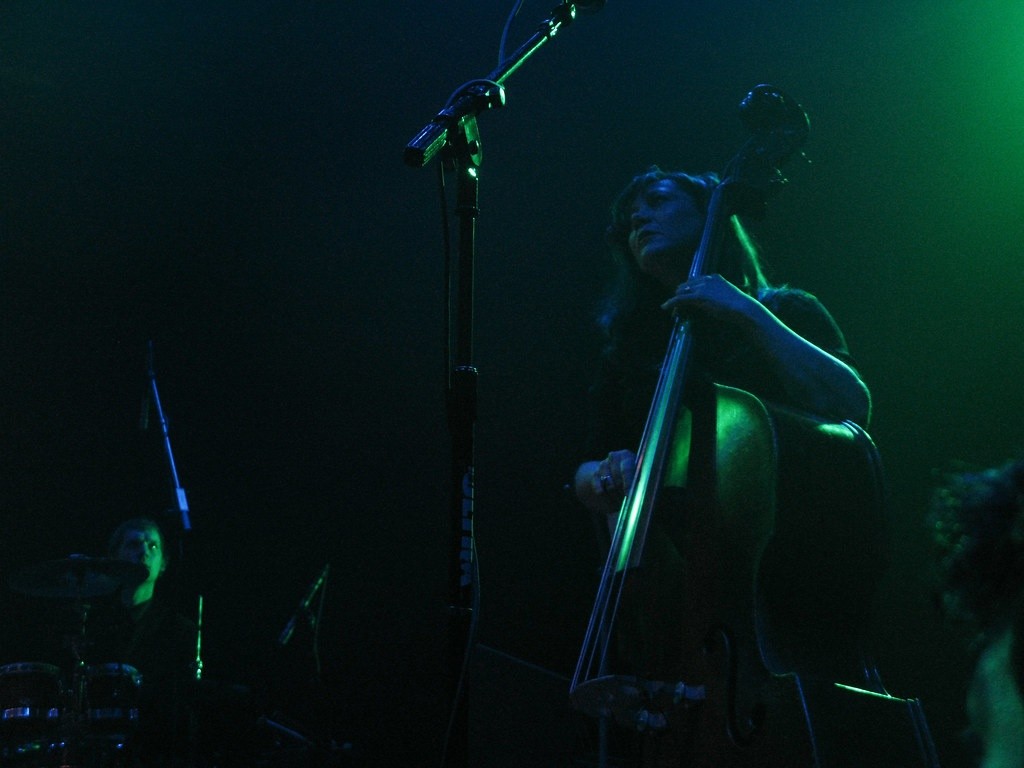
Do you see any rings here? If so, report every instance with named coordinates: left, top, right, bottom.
left=683, top=286, right=691, bottom=295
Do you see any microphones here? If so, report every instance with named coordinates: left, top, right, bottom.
left=139, top=339, right=154, bottom=430
left=277, top=564, right=334, bottom=646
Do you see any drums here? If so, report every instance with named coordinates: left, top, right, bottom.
left=2, top=659, right=75, bottom=751
left=71, top=661, right=144, bottom=748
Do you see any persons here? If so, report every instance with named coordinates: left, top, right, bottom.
left=958, top=465, right=1024, bottom=711
left=76, top=515, right=204, bottom=767
left=570, top=167, right=873, bottom=768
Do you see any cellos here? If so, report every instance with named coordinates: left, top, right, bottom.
left=592, top=82, right=941, bottom=768
